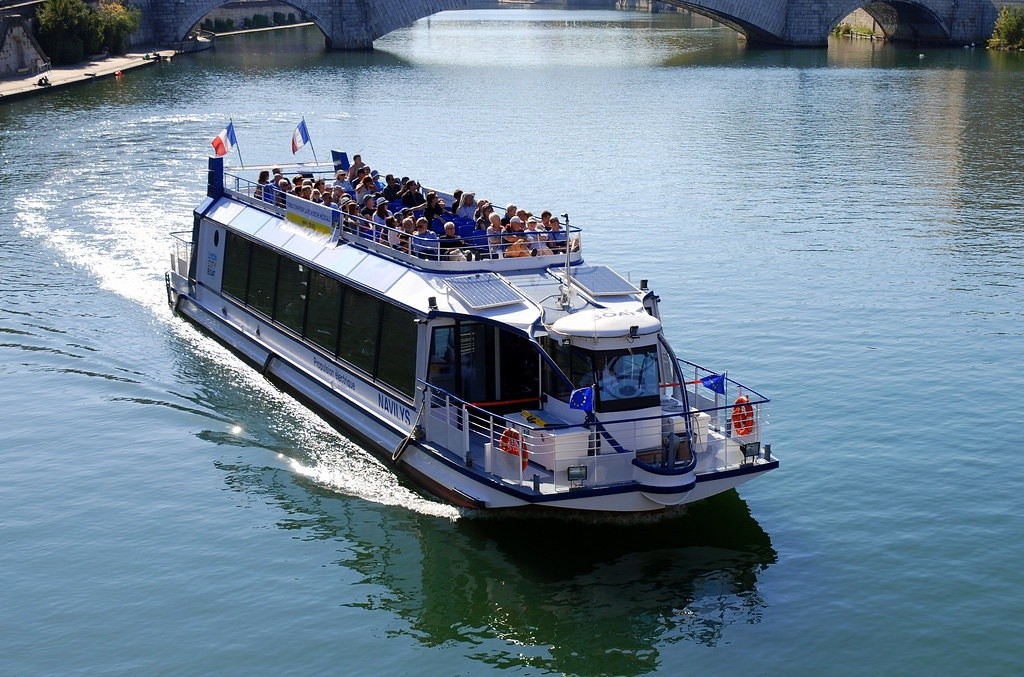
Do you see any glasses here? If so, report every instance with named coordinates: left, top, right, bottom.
left=340, top=174, right=346, bottom=176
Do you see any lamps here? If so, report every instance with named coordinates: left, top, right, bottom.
left=740, top=442, right=761, bottom=464
left=630, top=326, right=640, bottom=339
left=558, top=338, right=571, bottom=347
left=568, top=464, right=588, bottom=487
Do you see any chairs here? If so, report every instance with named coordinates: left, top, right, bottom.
left=265, top=182, right=488, bottom=252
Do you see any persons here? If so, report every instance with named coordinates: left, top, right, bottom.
left=239, top=21, right=245, bottom=29
left=38, top=75, right=51, bottom=86
left=143, top=47, right=159, bottom=60
left=254, top=154, right=570, bottom=260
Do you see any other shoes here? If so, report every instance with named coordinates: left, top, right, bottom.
left=529, top=247, right=537, bottom=256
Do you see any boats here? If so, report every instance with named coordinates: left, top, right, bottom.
left=164, top=150, right=779, bottom=512
left=173, top=29, right=216, bottom=53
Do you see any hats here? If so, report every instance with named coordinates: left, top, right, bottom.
left=371, top=170, right=380, bottom=180
left=528, top=217, right=537, bottom=224
left=510, top=216, right=524, bottom=224
left=339, top=197, right=352, bottom=209
left=337, top=170, right=346, bottom=176
left=376, top=196, right=389, bottom=207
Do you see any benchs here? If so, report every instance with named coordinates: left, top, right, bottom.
left=33, top=84, right=51, bottom=87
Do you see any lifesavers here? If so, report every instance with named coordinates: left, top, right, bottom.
left=500, top=428, right=528, bottom=472
left=732, top=396, right=753, bottom=435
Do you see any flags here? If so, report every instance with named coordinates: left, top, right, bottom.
left=292, top=119, right=311, bottom=155
left=570, top=386, right=594, bottom=409
left=212, top=121, right=238, bottom=157
left=700, top=373, right=726, bottom=395
